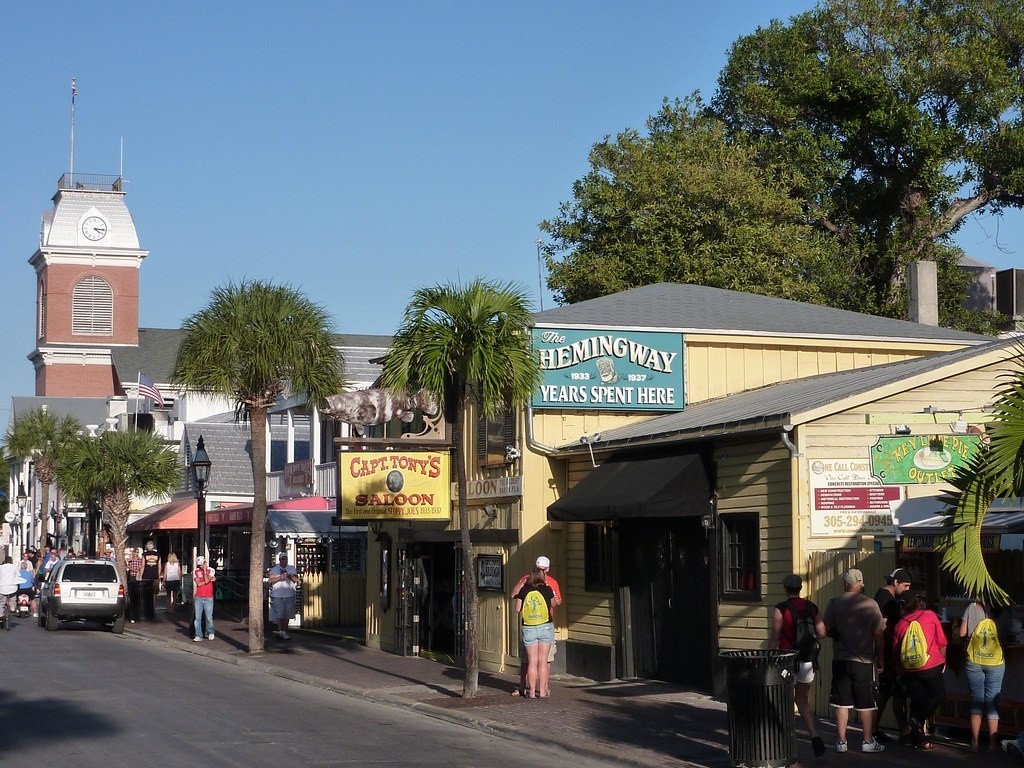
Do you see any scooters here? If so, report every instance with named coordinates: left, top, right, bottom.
left=16, top=591, right=30, bottom=617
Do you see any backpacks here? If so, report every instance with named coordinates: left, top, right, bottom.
left=900, top=620, right=930, bottom=669
left=522, top=589, right=549, bottom=625
left=783, top=600, right=821, bottom=672
left=966, top=618, right=1003, bottom=666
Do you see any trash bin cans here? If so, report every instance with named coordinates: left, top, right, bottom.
left=718, top=649, right=798, bottom=768
left=127, top=579, right=155, bottom=621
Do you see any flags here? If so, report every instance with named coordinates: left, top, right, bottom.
left=139, top=372, right=163, bottom=409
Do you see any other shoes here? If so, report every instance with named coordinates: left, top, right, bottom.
left=512, top=687, right=528, bottom=696
left=907, top=718, right=926, bottom=739
left=208, top=633, right=215, bottom=640
left=872, top=730, right=893, bottom=743
left=899, top=731, right=920, bottom=743
left=967, top=746, right=980, bottom=753
left=545, top=688, right=552, bottom=693
left=835, top=738, right=848, bottom=753
left=33, top=612, right=39, bottom=617
left=538, top=692, right=550, bottom=698
left=811, top=736, right=826, bottom=757
left=279, top=630, right=291, bottom=639
left=862, top=736, right=887, bottom=752
left=193, top=636, right=203, bottom=642
left=528, top=694, right=537, bottom=699
left=911, top=739, right=936, bottom=749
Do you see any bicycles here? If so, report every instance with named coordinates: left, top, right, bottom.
left=3, top=594, right=11, bottom=632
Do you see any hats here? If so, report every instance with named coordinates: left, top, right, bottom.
left=279, top=552, right=287, bottom=559
left=103, top=549, right=113, bottom=553
left=536, top=556, right=550, bottom=567
left=840, top=569, right=864, bottom=584
left=782, top=573, right=802, bottom=587
left=889, top=568, right=912, bottom=582
left=196, top=556, right=206, bottom=567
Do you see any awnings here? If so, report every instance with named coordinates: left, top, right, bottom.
left=547, top=442, right=714, bottom=520
left=126, top=499, right=199, bottom=530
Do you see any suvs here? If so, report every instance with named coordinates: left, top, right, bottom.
left=38, top=554, right=126, bottom=634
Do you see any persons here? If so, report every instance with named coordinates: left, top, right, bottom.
left=268, top=554, right=299, bottom=640
left=511, top=556, right=562, bottom=699
left=193, top=556, right=216, bottom=642
left=128, top=541, right=183, bottom=623
left=0, top=545, right=117, bottom=619
left=769, top=568, right=1006, bottom=758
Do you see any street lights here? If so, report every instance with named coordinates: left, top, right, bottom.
left=16, top=480, right=27, bottom=560
left=189, top=435, right=212, bottom=639
